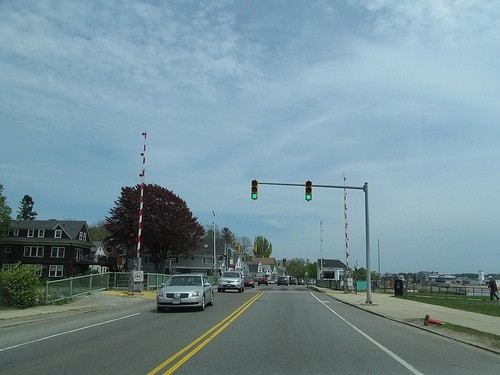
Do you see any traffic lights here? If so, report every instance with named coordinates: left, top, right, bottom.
left=304, top=181, right=313, bottom=202
left=250, top=179, right=259, bottom=200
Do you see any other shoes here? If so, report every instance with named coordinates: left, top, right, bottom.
left=491, top=299, right=493, bottom=301
left=497, top=298, right=499, bottom=301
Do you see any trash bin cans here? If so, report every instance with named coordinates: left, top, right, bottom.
left=394, top=279, right=407, bottom=298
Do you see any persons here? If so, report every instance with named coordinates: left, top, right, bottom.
left=487, top=276, right=499, bottom=301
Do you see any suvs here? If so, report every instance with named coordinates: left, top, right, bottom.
left=258, top=276, right=268, bottom=286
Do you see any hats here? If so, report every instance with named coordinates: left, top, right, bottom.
left=489, top=276, right=492, bottom=278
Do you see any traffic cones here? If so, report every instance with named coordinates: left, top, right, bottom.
left=423, top=314, right=447, bottom=326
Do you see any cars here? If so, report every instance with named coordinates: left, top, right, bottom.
left=289, top=279, right=298, bottom=285
left=156, top=274, right=214, bottom=311
left=244, top=278, right=255, bottom=288
left=307, top=280, right=316, bottom=285
left=277, top=276, right=289, bottom=286
left=217, top=271, right=245, bottom=293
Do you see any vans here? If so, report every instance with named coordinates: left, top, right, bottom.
left=298, top=279, right=306, bottom=285
